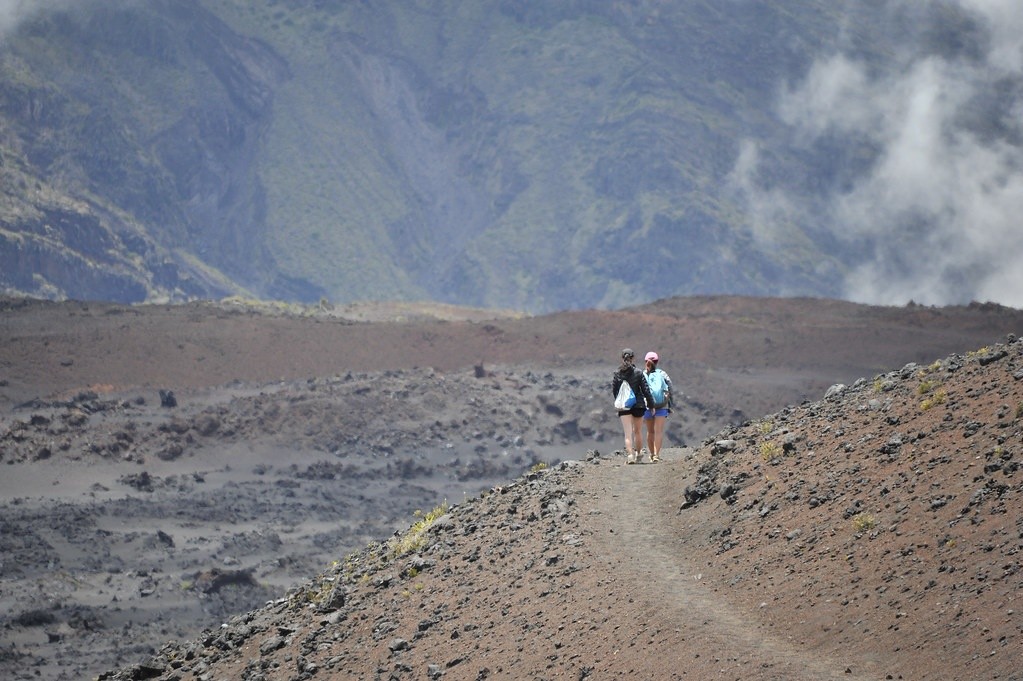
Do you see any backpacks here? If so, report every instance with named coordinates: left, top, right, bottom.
left=614, top=379, right=636, bottom=412
left=643, top=371, right=669, bottom=409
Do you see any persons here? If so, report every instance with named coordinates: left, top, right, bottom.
left=640, top=351, right=674, bottom=462
left=612, top=348, right=655, bottom=464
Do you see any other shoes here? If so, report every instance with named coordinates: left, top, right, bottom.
left=635, top=456, right=642, bottom=462
left=626, top=456, right=635, bottom=464
left=649, top=454, right=659, bottom=461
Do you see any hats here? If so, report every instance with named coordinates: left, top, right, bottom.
left=622, top=349, right=634, bottom=357
left=645, top=352, right=658, bottom=361
